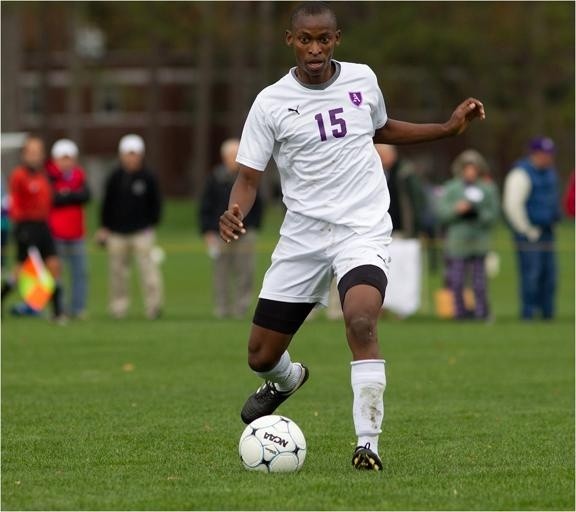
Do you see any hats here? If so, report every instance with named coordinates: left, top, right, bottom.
left=51, top=139, right=79, bottom=159
left=120, top=134, right=145, bottom=154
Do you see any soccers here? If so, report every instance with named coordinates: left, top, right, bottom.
left=238, top=414, right=306, bottom=473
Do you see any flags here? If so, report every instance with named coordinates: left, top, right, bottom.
left=13, top=246, right=56, bottom=311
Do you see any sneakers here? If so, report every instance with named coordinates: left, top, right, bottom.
left=352, top=442, right=383, bottom=470
left=241, top=362, right=309, bottom=424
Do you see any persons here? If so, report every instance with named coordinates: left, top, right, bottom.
left=96, top=134, right=164, bottom=319
left=427, top=150, right=503, bottom=319
left=1, top=133, right=69, bottom=325
left=373, top=129, right=404, bottom=239
left=217, top=5, right=485, bottom=470
left=503, top=136, right=564, bottom=318
left=45, top=138, right=92, bottom=320
left=198, top=140, right=265, bottom=319
